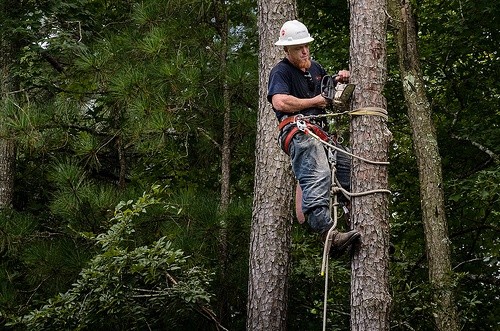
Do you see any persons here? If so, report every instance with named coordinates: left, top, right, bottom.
left=266, top=20, right=361, bottom=261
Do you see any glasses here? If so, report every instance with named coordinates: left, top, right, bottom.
left=304, top=70, right=315, bottom=92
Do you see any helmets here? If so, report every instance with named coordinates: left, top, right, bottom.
left=274, top=20, right=314, bottom=46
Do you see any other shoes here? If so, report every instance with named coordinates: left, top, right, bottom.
left=320, top=231, right=361, bottom=260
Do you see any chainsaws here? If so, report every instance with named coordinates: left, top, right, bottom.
left=321, top=74, right=356, bottom=111
